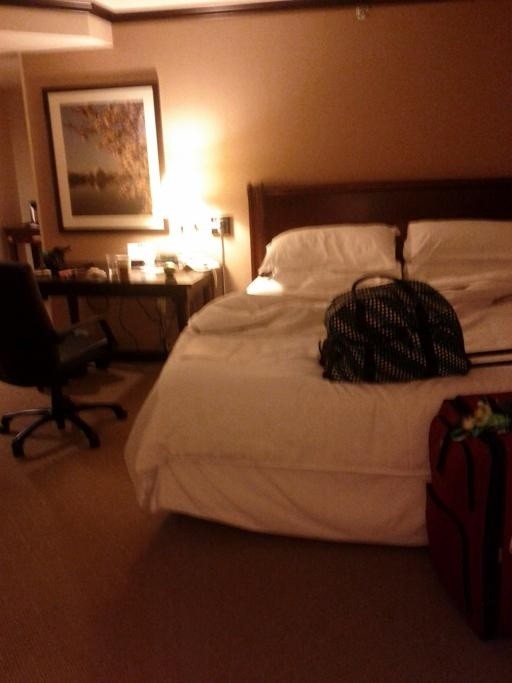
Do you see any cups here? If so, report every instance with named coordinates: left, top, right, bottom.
left=105, top=253, right=131, bottom=275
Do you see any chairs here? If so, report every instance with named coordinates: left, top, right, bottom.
left=1, top=257, right=134, bottom=462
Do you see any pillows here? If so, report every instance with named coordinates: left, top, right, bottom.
left=252, top=216, right=400, bottom=276
left=398, top=218, right=512, bottom=263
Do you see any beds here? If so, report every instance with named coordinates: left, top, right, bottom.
left=119, top=172, right=511, bottom=549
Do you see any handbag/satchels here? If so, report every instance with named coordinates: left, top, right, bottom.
left=319, top=271, right=472, bottom=384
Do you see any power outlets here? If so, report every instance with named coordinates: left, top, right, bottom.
left=219, top=215, right=233, bottom=236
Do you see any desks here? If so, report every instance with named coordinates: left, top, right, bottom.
left=22, top=256, right=219, bottom=349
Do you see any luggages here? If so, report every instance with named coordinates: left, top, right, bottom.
left=424, top=390, right=512, bottom=642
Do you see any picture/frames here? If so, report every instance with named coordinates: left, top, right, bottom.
left=38, top=77, right=176, bottom=236
